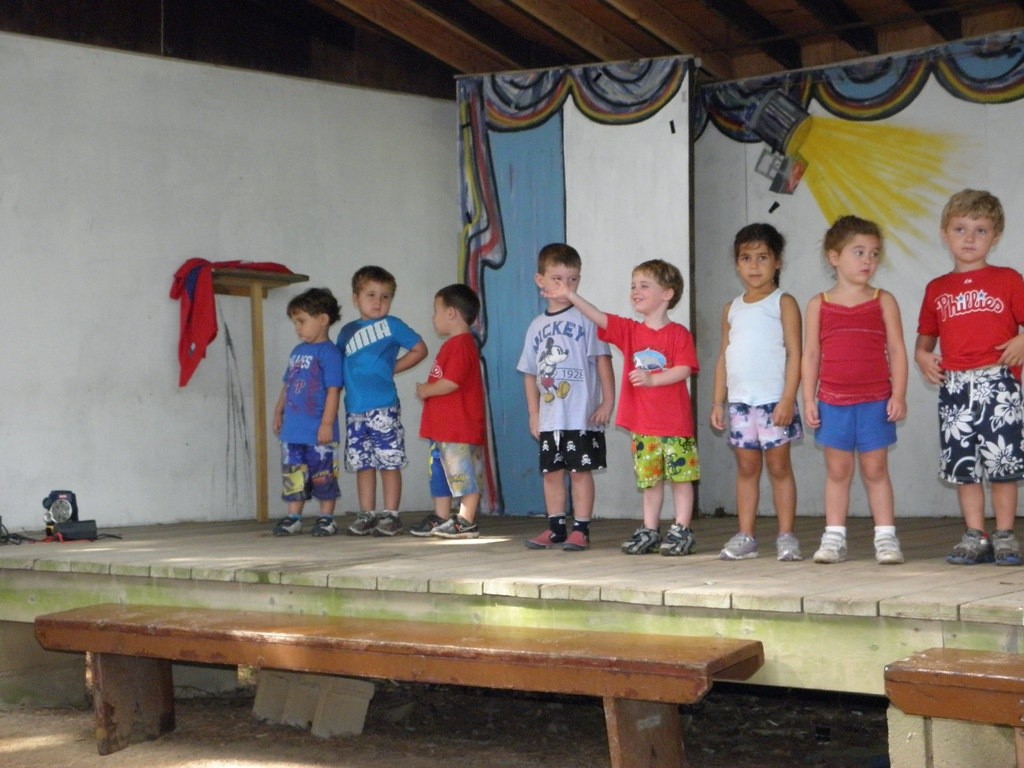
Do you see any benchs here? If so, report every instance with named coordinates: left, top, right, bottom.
left=35, top=611, right=1023, bottom=768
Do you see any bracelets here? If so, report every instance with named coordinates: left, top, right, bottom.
left=711, top=402, right=725, bottom=410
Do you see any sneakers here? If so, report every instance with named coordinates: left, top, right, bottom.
left=432, top=515, right=479, bottom=538
left=310, top=517, right=337, bottom=536
left=347, top=512, right=377, bottom=534
left=721, top=532, right=760, bottom=559
left=945, top=528, right=994, bottom=564
left=274, top=516, right=301, bottom=536
left=813, top=530, right=848, bottom=563
left=776, top=532, right=802, bottom=562
left=990, top=530, right=1023, bottom=565
left=373, top=512, right=403, bottom=536
left=660, top=524, right=695, bottom=554
left=621, top=525, right=662, bottom=554
left=411, top=512, right=449, bottom=536
left=874, top=532, right=903, bottom=564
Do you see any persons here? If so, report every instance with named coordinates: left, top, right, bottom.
left=709, top=224, right=803, bottom=561
left=914, top=188, right=1024, bottom=565
left=540, top=258, right=701, bottom=556
left=272, top=288, right=343, bottom=536
left=516, top=244, right=615, bottom=550
left=801, top=214, right=908, bottom=564
left=409, top=284, right=487, bottom=539
left=336, top=265, right=428, bottom=536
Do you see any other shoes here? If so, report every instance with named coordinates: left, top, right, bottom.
left=525, top=529, right=566, bottom=549
left=561, top=531, right=590, bottom=550
